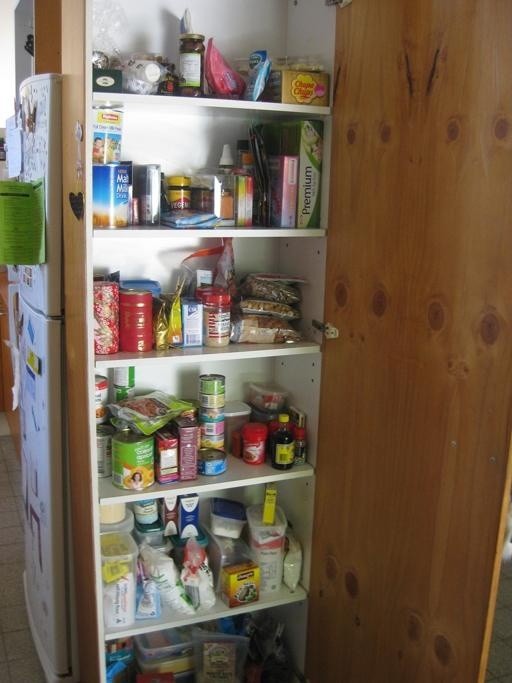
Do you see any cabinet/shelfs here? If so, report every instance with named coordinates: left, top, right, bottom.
left=32, top=3, right=512, bottom=682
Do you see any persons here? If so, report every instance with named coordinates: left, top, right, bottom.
left=129, top=471, right=149, bottom=490
left=93, top=136, right=105, bottom=159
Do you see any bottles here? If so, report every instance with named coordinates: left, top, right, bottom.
left=196, top=287, right=231, bottom=347
left=272, top=415, right=295, bottom=470
left=294, top=428, right=307, bottom=467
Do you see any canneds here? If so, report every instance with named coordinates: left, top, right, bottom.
left=111, top=432, right=156, bottom=490
left=178, top=34, right=205, bottom=97
left=113, top=367, right=135, bottom=387
left=120, top=287, right=153, bottom=353
left=92, top=103, right=125, bottom=168
left=94, top=374, right=109, bottom=424
left=198, top=374, right=227, bottom=476
left=92, top=161, right=133, bottom=229
left=167, top=175, right=191, bottom=211
left=112, top=386, right=135, bottom=403
left=202, top=292, right=231, bottom=347
left=196, top=286, right=225, bottom=303
left=96, top=424, right=115, bottom=478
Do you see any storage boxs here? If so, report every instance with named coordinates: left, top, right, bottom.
left=133, top=624, right=215, bottom=683
left=201, top=522, right=252, bottom=595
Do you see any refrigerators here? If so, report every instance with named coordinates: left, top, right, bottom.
left=7, top=76, right=66, bottom=557
